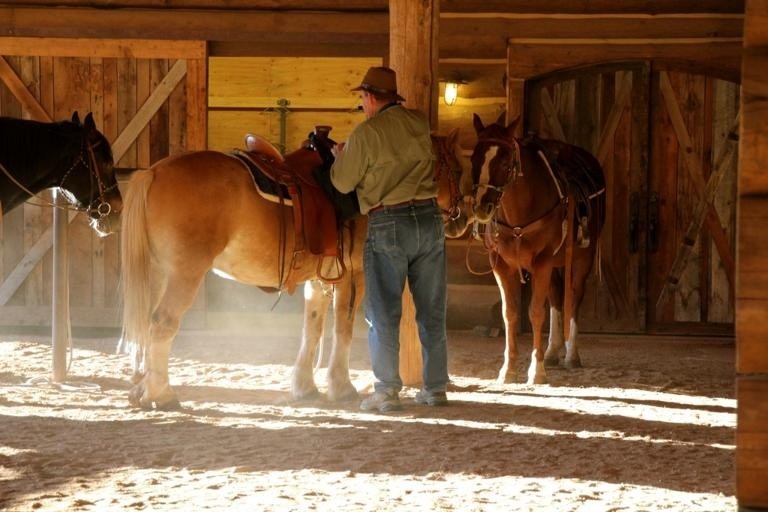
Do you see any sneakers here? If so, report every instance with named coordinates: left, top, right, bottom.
left=414, top=386, right=448, bottom=407
left=360, top=386, right=403, bottom=413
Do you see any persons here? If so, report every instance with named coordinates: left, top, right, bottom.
left=330, top=67, right=450, bottom=408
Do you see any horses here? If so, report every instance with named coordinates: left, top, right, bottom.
left=117, top=127, right=469, bottom=412
left=470, top=113, right=607, bottom=385
left=0, top=110, right=125, bottom=238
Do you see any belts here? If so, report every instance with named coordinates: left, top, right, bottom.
left=368, top=197, right=439, bottom=213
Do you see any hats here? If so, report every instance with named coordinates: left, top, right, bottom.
left=350, top=66, right=407, bottom=102
